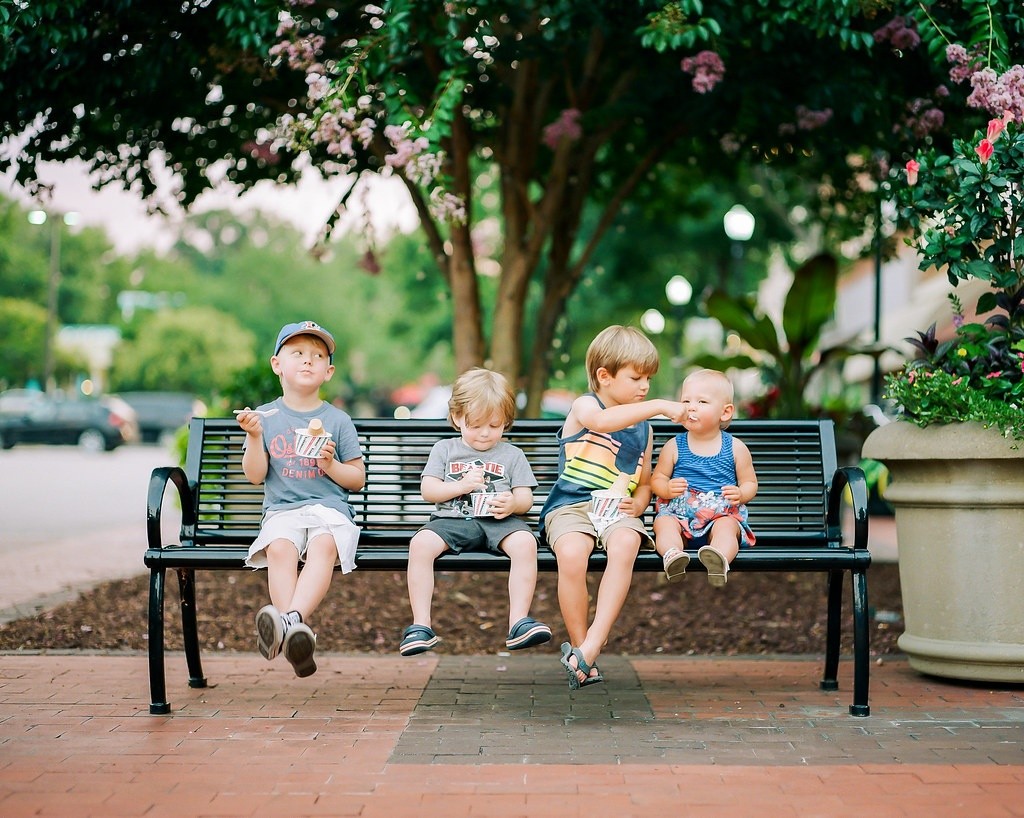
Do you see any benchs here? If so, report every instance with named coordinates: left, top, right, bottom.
left=143, top=415, right=877, bottom=720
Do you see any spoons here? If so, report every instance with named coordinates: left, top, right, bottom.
left=233, top=409, right=279, bottom=418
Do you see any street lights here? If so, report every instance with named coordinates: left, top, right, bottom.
left=27, top=209, right=82, bottom=393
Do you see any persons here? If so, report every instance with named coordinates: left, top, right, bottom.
left=650, top=369, right=758, bottom=587
left=236, top=321, right=365, bottom=677
left=538, top=325, right=690, bottom=690
left=400, top=369, right=552, bottom=656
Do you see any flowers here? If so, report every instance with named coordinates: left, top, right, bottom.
left=880, top=110, right=1023, bottom=452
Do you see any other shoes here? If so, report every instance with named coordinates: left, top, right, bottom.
left=663, top=548, right=690, bottom=583
left=255, top=605, right=293, bottom=660
left=282, top=623, right=317, bottom=678
left=698, top=545, right=729, bottom=586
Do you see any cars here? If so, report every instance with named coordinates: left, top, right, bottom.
left=0, top=386, right=208, bottom=452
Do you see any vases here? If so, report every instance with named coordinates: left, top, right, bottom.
left=862, top=419, right=1023, bottom=688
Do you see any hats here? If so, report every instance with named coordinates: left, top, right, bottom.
left=274, top=321, right=335, bottom=364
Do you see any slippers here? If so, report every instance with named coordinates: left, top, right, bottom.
left=560, top=640, right=589, bottom=689
left=569, top=662, right=603, bottom=688
left=505, top=616, right=551, bottom=650
left=399, top=624, right=439, bottom=657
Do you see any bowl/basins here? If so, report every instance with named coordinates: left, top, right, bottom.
left=470, top=492, right=500, bottom=516
left=296, top=429, right=332, bottom=458
left=591, top=489, right=629, bottom=518
